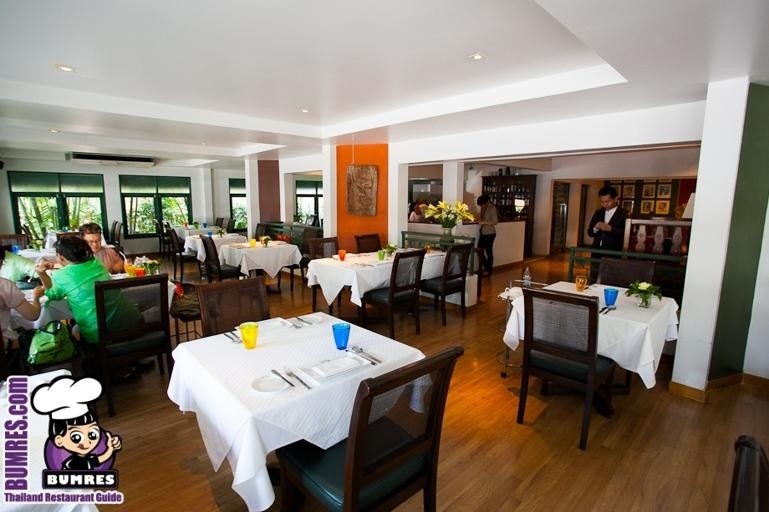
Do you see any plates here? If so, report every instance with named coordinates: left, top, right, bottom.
left=302, top=352, right=371, bottom=382
left=250, top=376, right=290, bottom=392
left=234, top=318, right=295, bottom=346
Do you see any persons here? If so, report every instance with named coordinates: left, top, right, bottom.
left=36, top=237, right=144, bottom=382
left=587, top=185, right=627, bottom=285
left=79, top=223, right=124, bottom=275
left=409, top=203, right=429, bottom=222
left=1, top=251, right=53, bottom=283
left=0, top=248, right=45, bottom=355
left=474, top=196, right=499, bottom=276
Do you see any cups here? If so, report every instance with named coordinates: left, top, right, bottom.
left=332, top=242, right=444, bottom=261
left=332, top=323, right=351, bottom=350
left=230, top=234, right=272, bottom=249
left=125, top=255, right=158, bottom=278
left=604, top=287, right=619, bottom=307
left=207, top=231, right=213, bottom=239
left=575, top=274, right=586, bottom=291
left=239, top=322, right=261, bottom=350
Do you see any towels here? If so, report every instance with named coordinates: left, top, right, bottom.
left=312, top=356, right=360, bottom=378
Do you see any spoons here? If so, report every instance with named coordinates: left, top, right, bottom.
left=605, top=305, right=619, bottom=316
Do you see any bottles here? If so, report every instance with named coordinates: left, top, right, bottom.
left=483, top=164, right=532, bottom=217
left=522, top=266, right=532, bottom=288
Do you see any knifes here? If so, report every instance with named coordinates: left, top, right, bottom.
left=345, top=348, right=377, bottom=368
left=293, top=315, right=314, bottom=326
left=270, top=368, right=295, bottom=389
left=599, top=306, right=607, bottom=314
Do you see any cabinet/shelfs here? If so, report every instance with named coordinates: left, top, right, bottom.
left=481, top=175, right=537, bottom=257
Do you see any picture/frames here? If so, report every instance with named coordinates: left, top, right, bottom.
left=611, top=179, right=679, bottom=217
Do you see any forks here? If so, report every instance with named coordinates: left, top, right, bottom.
left=284, top=369, right=311, bottom=391
left=284, top=315, right=303, bottom=330
left=354, top=345, right=381, bottom=362
left=223, top=332, right=240, bottom=345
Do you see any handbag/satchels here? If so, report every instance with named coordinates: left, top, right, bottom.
left=28, top=320, right=75, bottom=364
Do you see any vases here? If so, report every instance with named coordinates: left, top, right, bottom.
left=637, top=293, right=651, bottom=308
left=440, top=228, right=454, bottom=242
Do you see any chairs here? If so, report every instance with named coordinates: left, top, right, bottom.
left=354, top=233, right=382, bottom=253
left=595, top=257, right=656, bottom=289
left=292, top=346, right=463, bottom=512
left=517, top=287, right=617, bottom=451
left=196, top=276, right=271, bottom=336
left=361, top=248, right=427, bottom=341
left=728, top=435, right=768, bottom=512
left=420, top=242, right=473, bottom=326
left=0, top=217, right=323, bottom=289
left=94, top=274, right=174, bottom=418
left=308, top=237, right=342, bottom=315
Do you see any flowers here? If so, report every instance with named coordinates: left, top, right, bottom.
left=626, top=280, right=662, bottom=302
left=424, top=200, right=475, bottom=228
